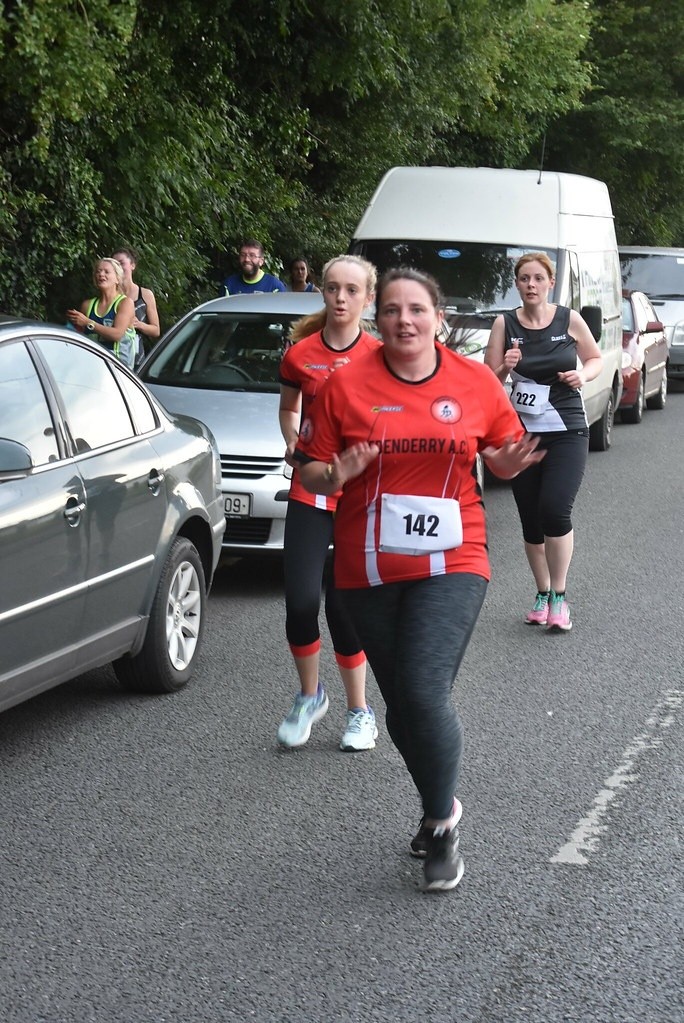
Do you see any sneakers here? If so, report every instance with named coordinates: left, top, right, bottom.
left=422, top=825, right=465, bottom=890
left=277, top=681, right=330, bottom=747
left=339, top=704, right=378, bottom=751
left=410, top=795, right=463, bottom=856
left=545, top=588, right=572, bottom=632
left=525, top=593, right=549, bottom=624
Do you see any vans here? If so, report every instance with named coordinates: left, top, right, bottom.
left=344, top=165, right=625, bottom=485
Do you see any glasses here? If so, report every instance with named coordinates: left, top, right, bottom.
left=239, top=252, right=262, bottom=259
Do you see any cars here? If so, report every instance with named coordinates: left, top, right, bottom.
left=617, top=289, right=671, bottom=423
left=0, top=310, right=228, bottom=717
left=134, top=291, right=484, bottom=557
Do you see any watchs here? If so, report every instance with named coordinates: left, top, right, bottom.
left=85, top=320, right=96, bottom=331
left=323, top=463, right=339, bottom=487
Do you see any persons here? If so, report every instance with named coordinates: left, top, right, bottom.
left=68, top=258, right=137, bottom=373
left=273, top=256, right=387, bottom=754
left=109, top=246, right=161, bottom=375
left=219, top=239, right=287, bottom=299
left=294, top=268, right=547, bottom=892
left=483, top=251, right=605, bottom=634
left=287, top=256, right=322, bottom=294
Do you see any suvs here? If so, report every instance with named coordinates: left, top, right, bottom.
left=618, top=246, right=684, bottom=380
